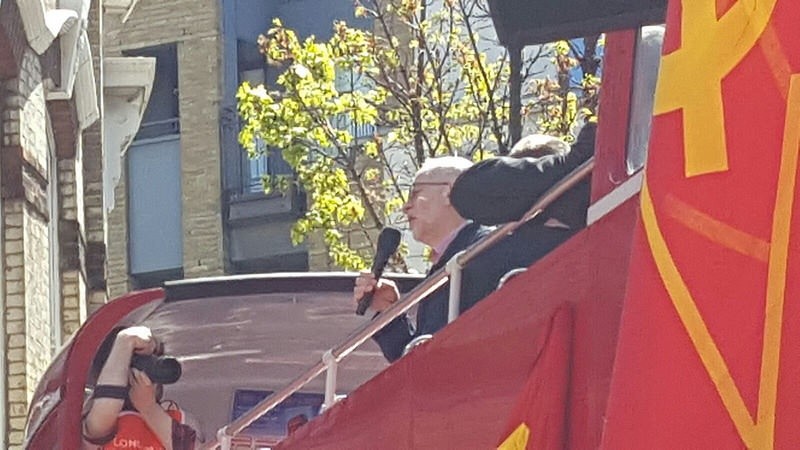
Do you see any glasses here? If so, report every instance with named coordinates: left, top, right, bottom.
left=408, top=182, right=449, bottom=199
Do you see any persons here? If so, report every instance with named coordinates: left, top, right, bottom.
left=78, top=325, right=201, bottom=450
left=353, top=121, right=596, bottom=366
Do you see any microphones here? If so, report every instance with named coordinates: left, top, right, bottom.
left=355, top=227, right=402, bottom=315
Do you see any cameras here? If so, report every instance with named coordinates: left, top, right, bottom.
left=130, top=353, right=181, bottom=384
